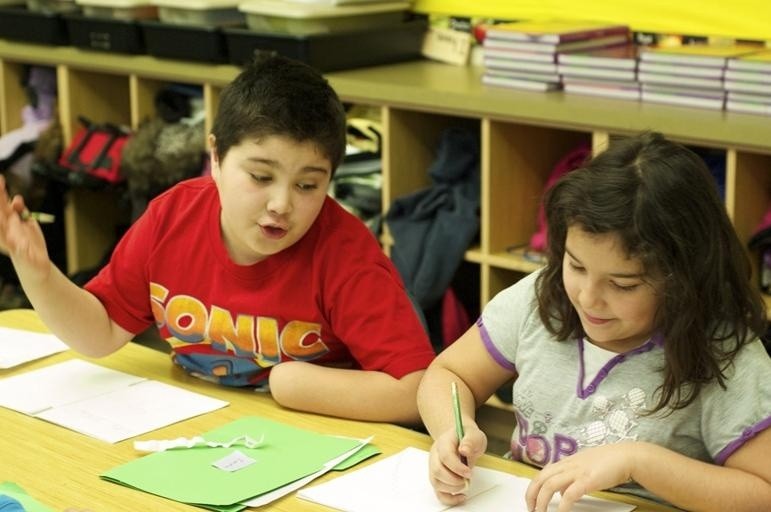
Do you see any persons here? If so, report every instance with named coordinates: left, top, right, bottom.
left=0, top=56, right=436, bottom=423
left=415, top=134, right=771, bottom=512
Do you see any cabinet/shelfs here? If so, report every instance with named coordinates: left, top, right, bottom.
left=0, top=41, right=771, bottom=408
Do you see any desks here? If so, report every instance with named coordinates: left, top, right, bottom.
left=2, top=307, right=681, bottom=512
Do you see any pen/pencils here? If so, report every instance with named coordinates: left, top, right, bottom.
left=451, top=380, right=474, bottom=485
left=19, top=209, right=55, bottom=222
